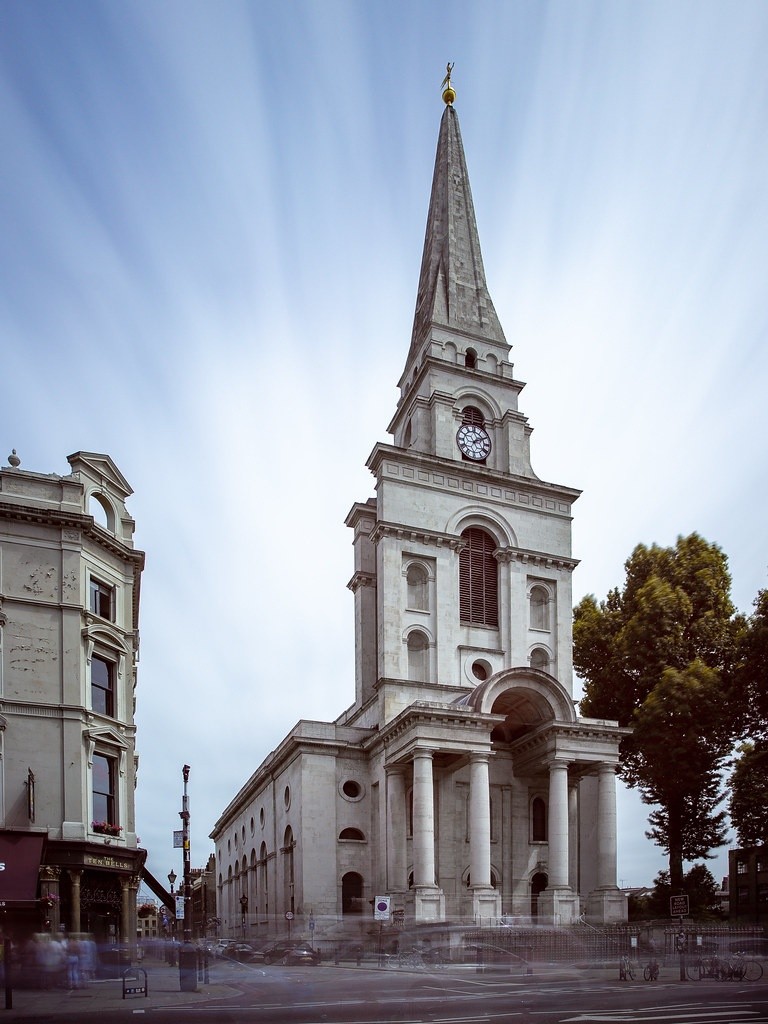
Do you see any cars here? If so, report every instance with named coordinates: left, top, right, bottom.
left=195, top=939, right=215, bottom=956
left=221, top=942, right=254, bottom=961
left=211, top=939, right=237, bottom=960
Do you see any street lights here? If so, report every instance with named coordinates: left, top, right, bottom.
left=239, top=893, right=248, bottom=941
left=168, top=869, right=177, bottom=967
left=179, top=765, right=198, bottom=992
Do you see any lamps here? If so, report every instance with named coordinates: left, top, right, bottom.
left=456, top=424, right=492, bottom=460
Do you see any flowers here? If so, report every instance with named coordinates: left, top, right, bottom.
left=91, top=819, right=122, bottom=831
left=139, top=904, right=157, bottom=913
left=40, top=892, right=61, bottom=907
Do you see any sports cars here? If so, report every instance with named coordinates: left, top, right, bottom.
left=263, top=941, right=320, bottom=966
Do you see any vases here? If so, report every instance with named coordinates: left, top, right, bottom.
left=138, top=910, right=155, bottom=918
left=44, top=904, right=53, bottom=909
left=93, top=825, right=119, bottom=837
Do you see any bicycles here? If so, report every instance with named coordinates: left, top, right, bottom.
left=387, top=946, right=450, bottom=972
left=618, top=953, right=636, bottom=981
left=642, top=958, right=660, bottom=981
left=686, top=948, right=763, bottom=982
